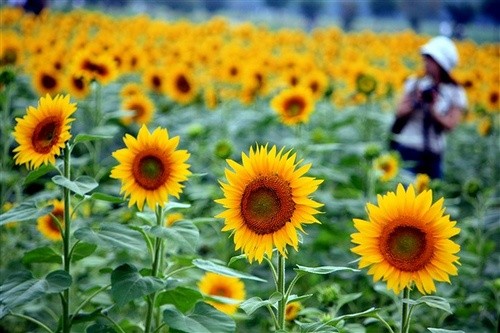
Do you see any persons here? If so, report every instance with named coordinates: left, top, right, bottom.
left=388, top=35, right=468, bottom=181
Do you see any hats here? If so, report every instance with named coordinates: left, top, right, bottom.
left=419, top=36, right=458, bottom=72
